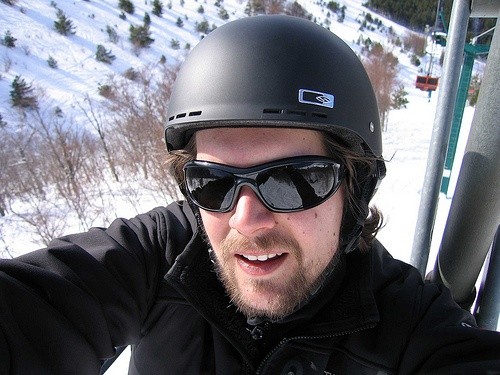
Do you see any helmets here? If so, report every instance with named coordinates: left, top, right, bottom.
left=161, top=11, right=383, bottom=158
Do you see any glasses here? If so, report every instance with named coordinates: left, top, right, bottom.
left=176, top=154, right=350, bottom=215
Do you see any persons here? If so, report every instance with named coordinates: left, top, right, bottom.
left=1, top=15, right=499, bottom=375
